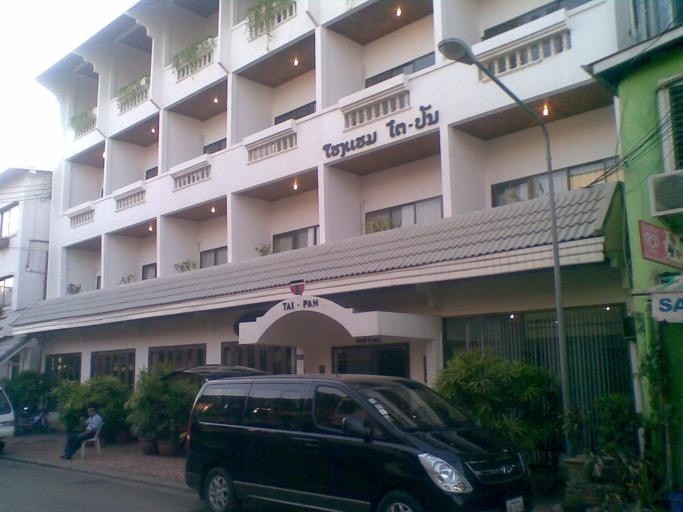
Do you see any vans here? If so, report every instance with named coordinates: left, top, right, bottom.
left=154, top=363, right=534, bottom=511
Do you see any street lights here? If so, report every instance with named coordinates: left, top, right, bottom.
left=437, top=38, right=579, bottom=456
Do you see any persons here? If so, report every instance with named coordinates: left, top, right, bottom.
left=58, top=406, right=102, bottom=460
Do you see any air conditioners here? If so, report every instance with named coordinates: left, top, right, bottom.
left=648, top=169, right=683, bottom=217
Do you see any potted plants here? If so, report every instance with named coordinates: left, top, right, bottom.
left=50, top=361, right=200, bottom=457
left=558, top=391, right=657, bottom=484
